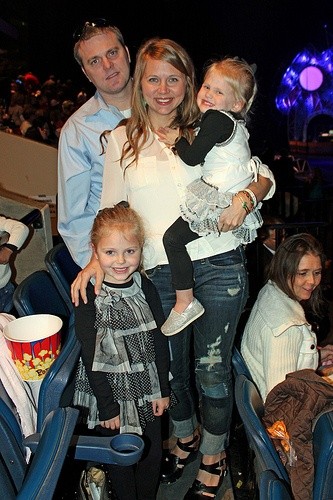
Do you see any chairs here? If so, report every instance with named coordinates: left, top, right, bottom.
left=0, top=199, right=332, bottom=499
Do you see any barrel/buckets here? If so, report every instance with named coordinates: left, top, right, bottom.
left=3, top=314, right=63, bottom=382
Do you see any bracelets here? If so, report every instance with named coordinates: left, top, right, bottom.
left=238, top=189, right=254, bottom=211
left=235, top=193, right=251, bottom=215
left=243, top=188, right=257, bottom=209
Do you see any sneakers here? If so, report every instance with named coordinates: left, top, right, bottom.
left=161, top=297, right=205, bottom=336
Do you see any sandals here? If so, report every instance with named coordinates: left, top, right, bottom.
left=183, top=458, right=228, bottom=500
left=160, top=433, right=201, bottom=485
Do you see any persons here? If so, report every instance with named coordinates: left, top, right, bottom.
left=154, top=57, right=263, bottom=336
left=239, top=233, right=333, bottom=405
left=0, top=47, right=73, bottom=137
left=55, top=99, right=76, bottom=145
left=238, top=218, right=287, bottom=308
left=25, top=117, right=51, bottom=144
left=57, top=18, right=147, bottom=286
left=0, top=214, right=30, bottom=318
left=74, top=91, right=90, bottom=113
left=69, top=37, right=276, bottom=498
left=74, top=201, right=178, bottom=500
